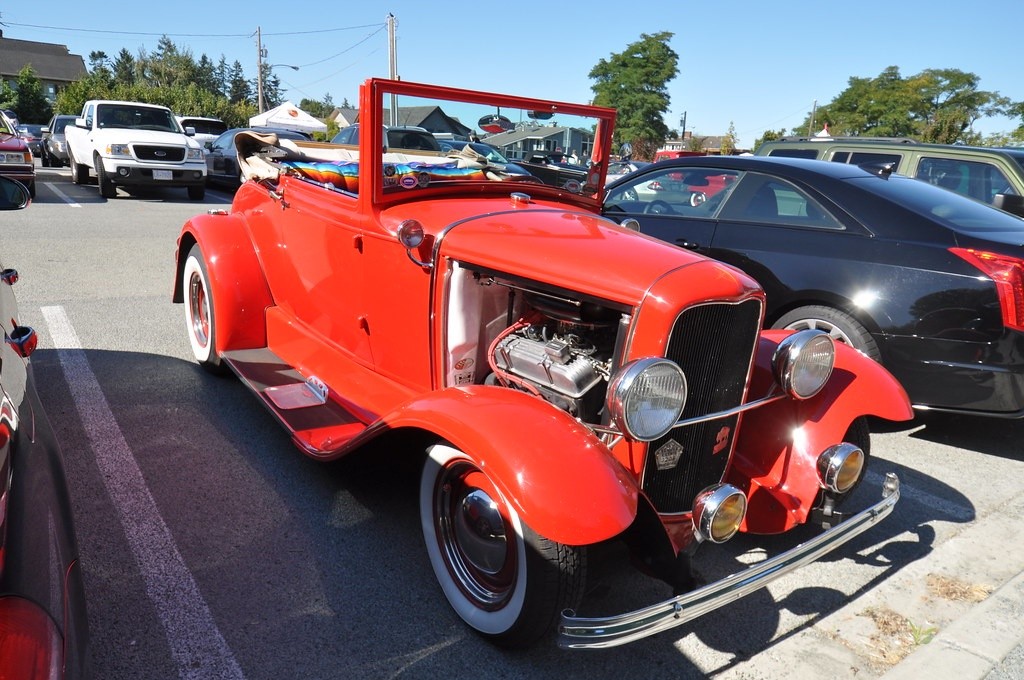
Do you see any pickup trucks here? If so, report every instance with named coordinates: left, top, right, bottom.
left=63, top=99, right=208, bottom=201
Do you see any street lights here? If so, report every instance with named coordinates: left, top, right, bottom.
left=256, top=26, right=300, bottom=114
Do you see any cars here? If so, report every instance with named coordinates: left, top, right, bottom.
left=177, top=115, right=228, bottom=153
left=0, top=170, right=94, bottom=680
left=17, top=124, right=44, bottom=156
left=599, top=159, right=674, bottom=196
left=653, top=150, right=734, bottom=208
left=39, top=114, right=82, bottom=168
left=0, top=109, right=36, bottom=198
left=329, top=123, right=441, bottom=152
left=596, top=152, right=1023, bottom=424
left=435, top=138, right=531, bottom=178
left=202, top=126, right=313, bottom=193
left=170, top=77, right=918, bottom=653
left=507, top=148, right=589, bottom=190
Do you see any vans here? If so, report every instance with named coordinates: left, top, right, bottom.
left=754, top=142, right=1024, bottom=216
